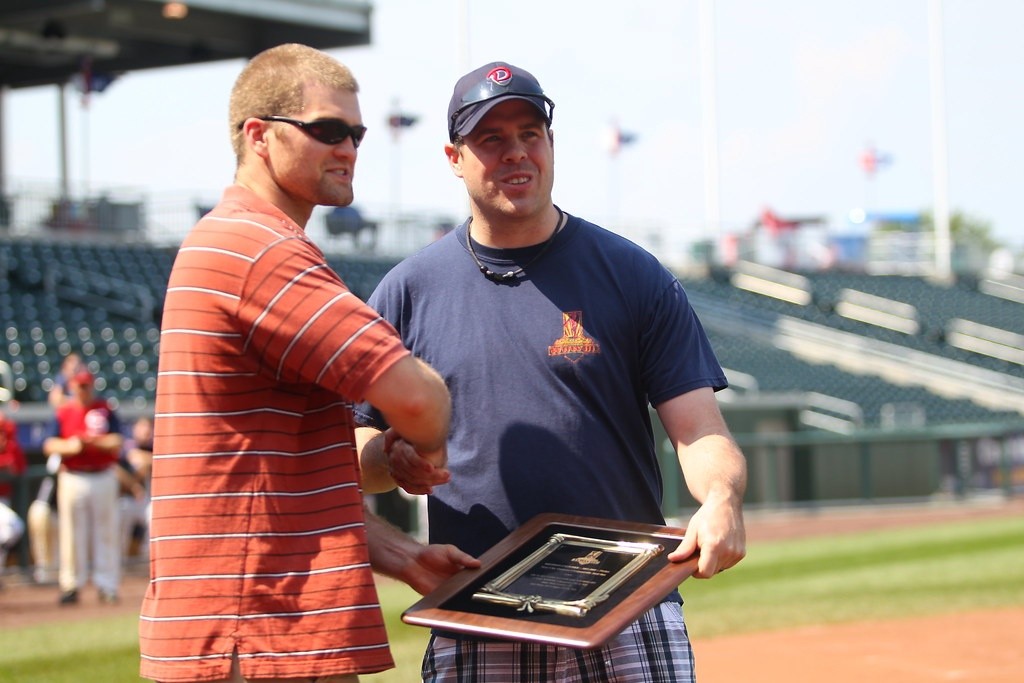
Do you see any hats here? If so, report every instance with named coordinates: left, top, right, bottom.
left=446, top=61, right=553, bottom=143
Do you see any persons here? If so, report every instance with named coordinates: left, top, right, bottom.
left=347, top=54, right=752, bottom=683
left=42, top=368, right=126, bottom=603
left=134, top=40, right=458, bottom=683
left=1, top=355, right=152, bottom=556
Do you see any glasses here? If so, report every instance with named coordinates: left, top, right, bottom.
left=238, top=117, right=366, bottom=148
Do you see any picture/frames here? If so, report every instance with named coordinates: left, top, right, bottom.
left=401, top=512, right=699, bottom=652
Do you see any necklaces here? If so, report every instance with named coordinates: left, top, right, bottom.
left=465, top=201, right=563, bottom=280
